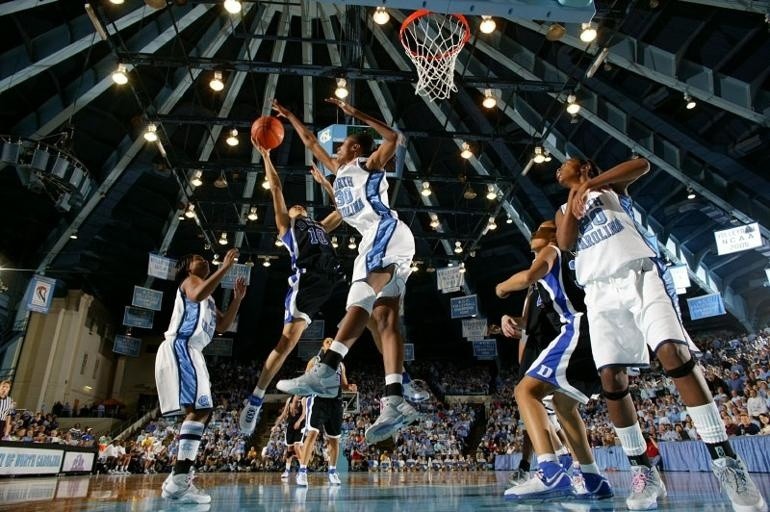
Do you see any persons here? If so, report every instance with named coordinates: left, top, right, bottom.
left=495, top=219, right=616, bottom=502
left=271, top=98, right=415, bottom=444
left=100, top=327, right=769, bottom=478
left=154, top=246, right=247, bottom=504
left=1, top=380, right=121, bottom=479
left=239, top=136, right=430, bottom=435
left=555, top=155, right=767, bottom=510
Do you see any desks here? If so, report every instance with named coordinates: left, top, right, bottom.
left=493, top=434, right=769, bottom=473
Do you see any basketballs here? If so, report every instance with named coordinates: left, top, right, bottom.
left=250, top=115, right=285, bottom=150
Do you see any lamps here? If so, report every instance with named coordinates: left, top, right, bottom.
left=83, top=1, right=636, bottom=273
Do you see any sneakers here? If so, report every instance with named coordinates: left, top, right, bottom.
left=281, top=470, right=289, bottom=477
left=239, top=396, right=265, bottom=433
left=329, top=473, right=341, bottom=484
left=162, top=468, right=211, bottom=503
left=295, top=469, right=309, bottom=486
left=365, top=395, right=418, bottom=443
left=710, top=454, right=767, bottom=512
left=400, top=372, right=430, bottom=403
left=278, top=364, right=341, bottom=398
left=626, top=465, right=668, bottom=511
left=504, top=456, right=614, bottom=500
